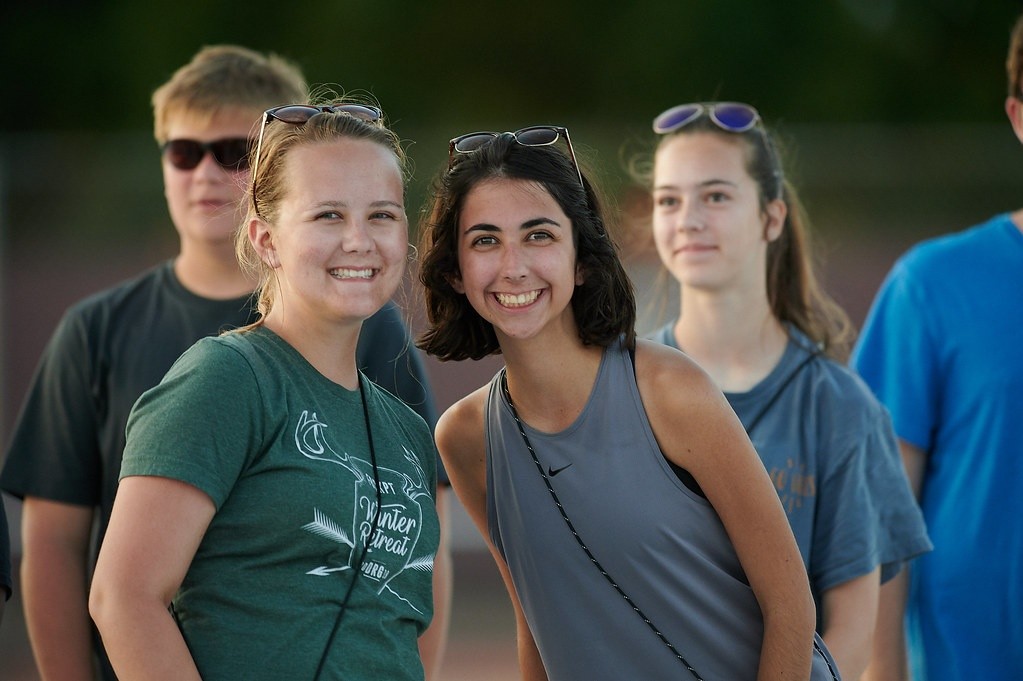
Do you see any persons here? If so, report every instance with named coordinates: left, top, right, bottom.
left=0, top=45, right=458, bottom=681
left=416, top=126, right=840, bottom=681
left=88, top=98, right=441, bottom=681
left=641, top=103, right=934, bottom=681
left=847, top=16, right=1023, bottom=681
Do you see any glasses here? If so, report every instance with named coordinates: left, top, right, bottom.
left=652, top=103, right=770, bottom=162
left=252, top=103, right=383, bottom=217
left=448, top=126, right=582, bottom=190
left=157, top=137, right=260, bottom=172
